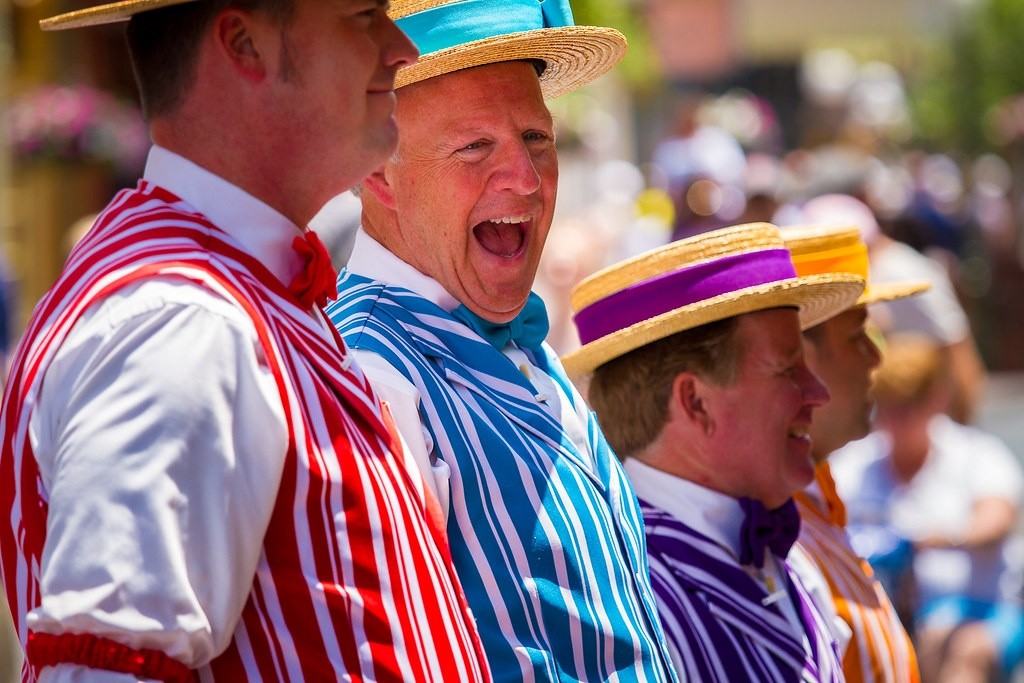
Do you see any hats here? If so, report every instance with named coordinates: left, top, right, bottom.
left=563, top=221, right=865, bottom=381
left=393, top=0, right=628, bottom=95
left=38, top=0, right=460, bottom=31
left=785, top=227, right=934, bottom=309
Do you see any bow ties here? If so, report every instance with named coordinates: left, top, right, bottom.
left=289, top=232, right=338, bottom=310
left=814, top=460, right=846, bottom=527
left=450, top=291, right=549, bottom=353
left=739, top=496, right=802, bottom=570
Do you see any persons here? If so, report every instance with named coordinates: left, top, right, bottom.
left=328, top=0, right=669, bottom=683
left=563, top=98, right=1024, bottom=682
left=3, top=1, right=494, bottom=682
